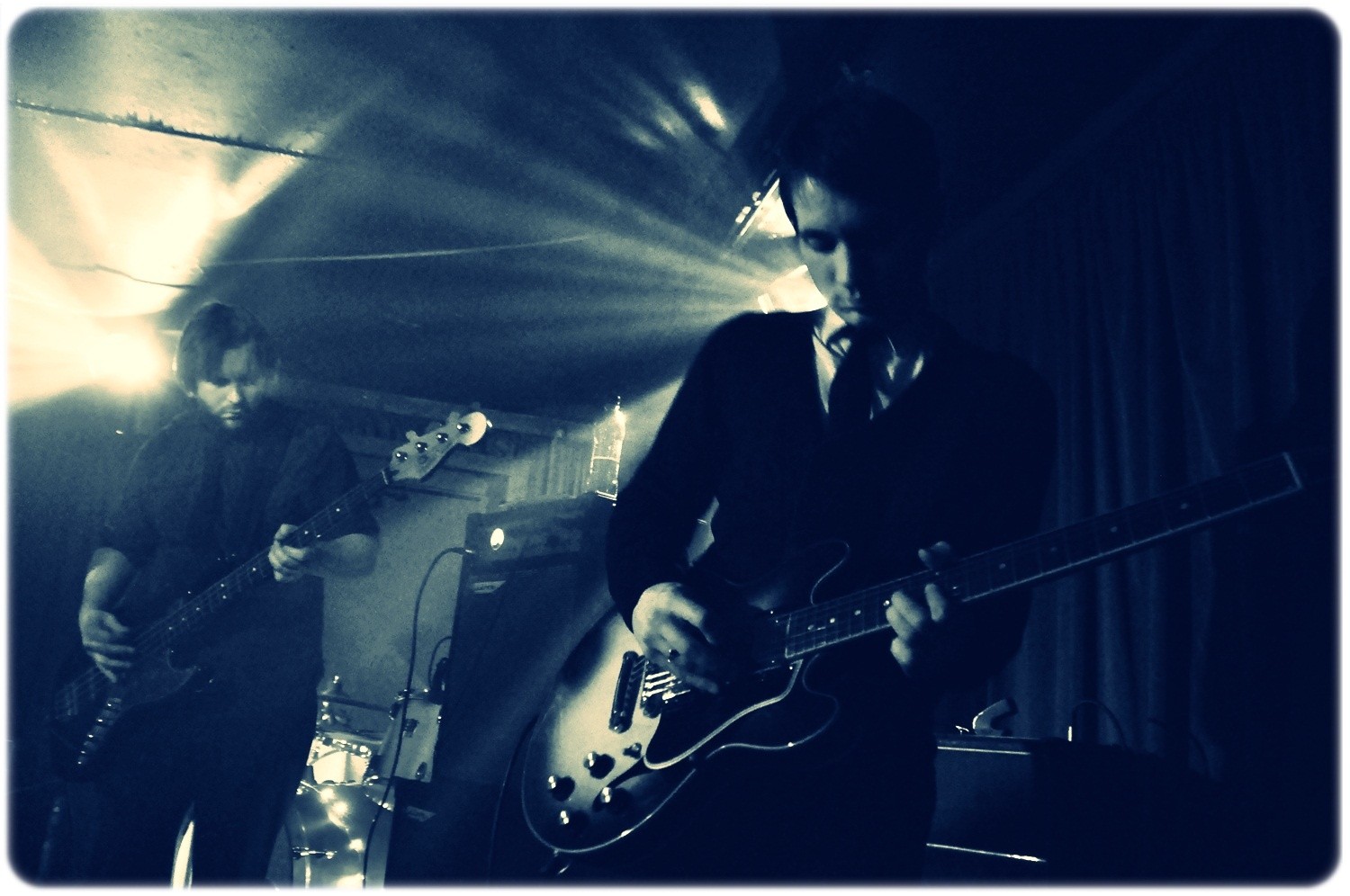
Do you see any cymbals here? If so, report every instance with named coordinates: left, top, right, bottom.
left=315, top=691, right=390, bottom=713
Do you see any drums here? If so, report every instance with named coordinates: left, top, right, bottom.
left=267, top=785, right=395, bottom=887
left=300, top=729, right=372, bottom=789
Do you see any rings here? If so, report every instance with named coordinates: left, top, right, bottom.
left=668, top=650, right=680, bottom=661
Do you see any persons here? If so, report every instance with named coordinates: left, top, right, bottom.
left=79, top=299, right=378, bottom=880
left=602, top=93, right=1057, bottom=883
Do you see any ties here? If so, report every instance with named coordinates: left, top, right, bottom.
left=828, top=325, right=892, bottom=434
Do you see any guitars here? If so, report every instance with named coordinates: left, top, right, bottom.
left=59, top=412, right=493, bottom=776
left=520, top=439, right=1338, bottom=854
